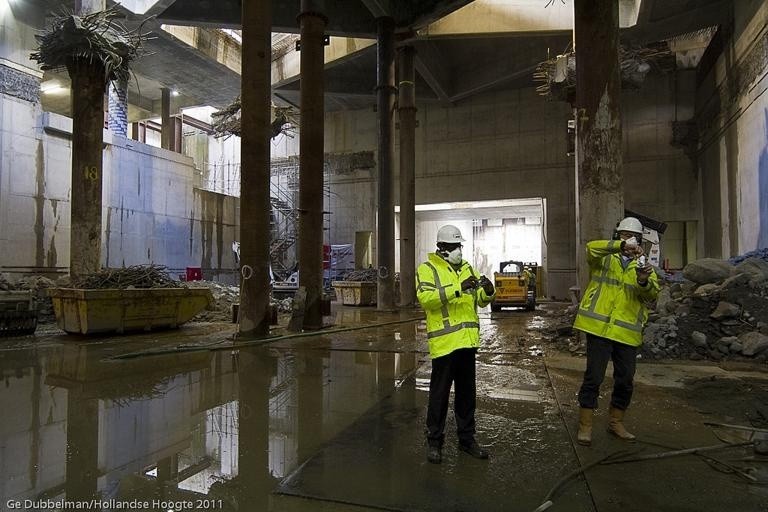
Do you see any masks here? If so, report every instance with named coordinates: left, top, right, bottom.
left=625, top=237, right=638, bottom=255
left=449, top=247, right=462, bottom=265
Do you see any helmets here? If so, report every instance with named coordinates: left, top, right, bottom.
left=437, top=225, right=465, bottom=244
left=616, top=217, right=643, bottom=234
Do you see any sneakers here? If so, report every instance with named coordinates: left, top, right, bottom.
left=428, top=447, right=442, bottom=464
left=462, top=443, right=489, bottom=459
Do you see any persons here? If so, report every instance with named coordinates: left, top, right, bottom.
left=412, top=223, right=496, bottom=462
left=570, top=215, right=662, bottom=446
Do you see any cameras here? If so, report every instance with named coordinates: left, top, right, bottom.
left=636, top=254, right=645, bottom=268
left=475, top=278, right=483, bottom=290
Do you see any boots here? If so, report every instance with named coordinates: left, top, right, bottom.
left=607, top=406, right=636, bottom=440
left=578, top=408, right=593, bottom=446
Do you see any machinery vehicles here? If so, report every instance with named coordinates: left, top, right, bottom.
left=489, top=258, right=534, bottom=308
